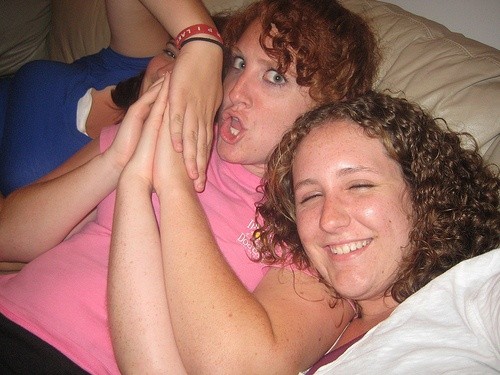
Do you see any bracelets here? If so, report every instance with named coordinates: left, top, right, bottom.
left=175, top=24, right=224, bottom=48
left=179, top=37, right=224, bottom=53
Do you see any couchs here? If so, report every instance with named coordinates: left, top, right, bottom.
left=0, top=0, right=500, bottom=180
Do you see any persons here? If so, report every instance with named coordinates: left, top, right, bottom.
left=1, top=1, right=224, bottom=273
left=107, top=68, right=500, bottom=375
left=1, top=0, right=383, bottom=375
